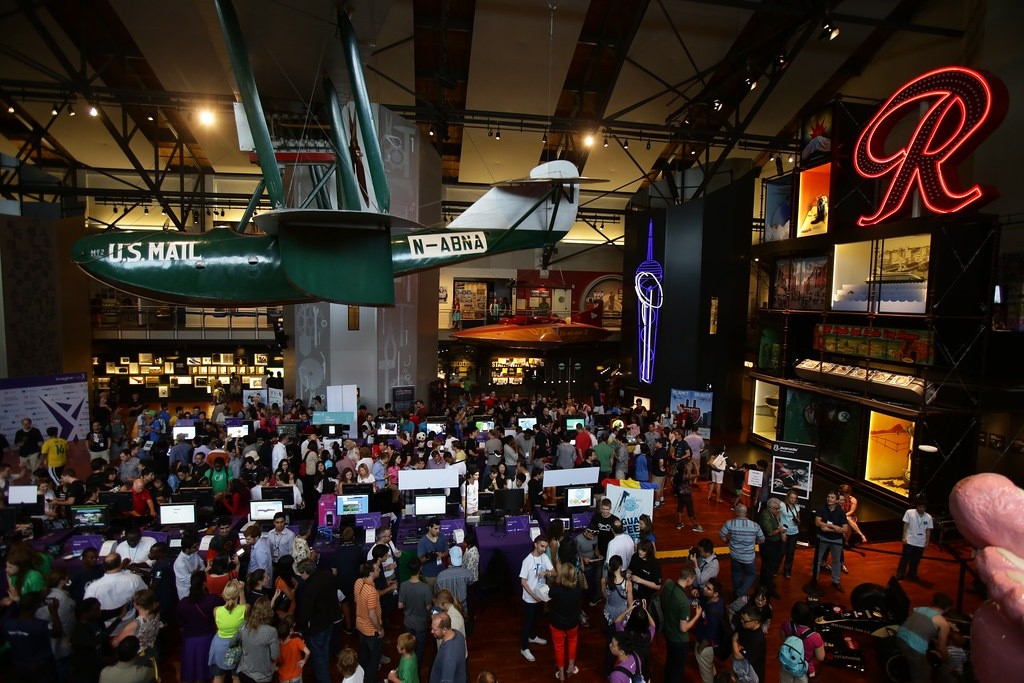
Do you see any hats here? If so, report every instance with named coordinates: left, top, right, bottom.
left=449, top=547, right=463, bottom=566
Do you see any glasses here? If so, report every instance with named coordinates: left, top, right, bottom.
left=740, top=617, right=757, bottom=624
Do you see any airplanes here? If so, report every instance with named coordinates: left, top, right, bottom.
left=67, top=0, right=611, bottom=310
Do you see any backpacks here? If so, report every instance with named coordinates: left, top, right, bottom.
left=615, top=652, right=646, bottom=683
left=777, top=620, right=814, bottom=677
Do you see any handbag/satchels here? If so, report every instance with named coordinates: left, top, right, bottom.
left=707, top=451, right=728, bottom=471
left=224, top=626, right=246, bottom=667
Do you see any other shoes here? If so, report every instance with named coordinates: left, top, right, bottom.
left=692, top=525, right=706, bottom=532
left=659, top=499, right=665, bottom=505
left=716, top=499, right=722, bottom=503
left=770, top=590, right=781, bottom=599
left=908, top=572, right=921, bottom=582
left=341, top=624, right=351, bottom=633
left=653, top=502, right=660, bottom=509
left=380, top=655, right=390, bottom=663
left=895, top=572, right=904, bottom=580
left=833, top=579, right=845, bottom=593
left=579, top=613, right=590, bottom=629
left=842, top=564, right=850, bottom=575
left=708, top=495, right=711, bottom=500
left=822, top=561, right=832, bottom=572
left=677, top=522, right=684, bottom=530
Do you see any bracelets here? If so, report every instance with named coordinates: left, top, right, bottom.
left=547, top=569, right=552, bottom=577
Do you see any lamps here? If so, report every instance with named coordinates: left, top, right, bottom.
left=820, top=12, right=839, bottom=39
left=744, top=72, right=756, bottom=90
left=146, top=109, right=156, bottom=120
left=712, top=96, right=725, bottom=112
left=52, top=102, right=60, bottom=116
left=429, top=118, right=793, bottom=163
left=444, top=212, right=454, bottom=223
left=113, top=203, right=259, bottom=216
left=592, top=221, right=606, bottom=230
left=8, top=96, right=16, bottom=113
left=66, top=101, right=75, bottom=116
left=775, top=50, right=787, bottom=71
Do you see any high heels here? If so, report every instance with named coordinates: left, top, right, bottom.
left=566, top=667, right=578, bottom=678
left=555, top=670, right=567, bottom=682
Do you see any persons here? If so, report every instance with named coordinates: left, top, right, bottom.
left=455, top=288, right=472, bottom=298
left=489, top=297, right=510, bottom=322
left=800, top=195, right=828, bottom=234
left=540, top=296, right=549, bottom=309
left=335, top=645, right=365, bottom=683
left=452, top=299, right=461, bottom=326
left=609, top=290, right=615, bottom=311
left=545, top=563, right=581, bottom=683
left=896, top=497, right=934, bottom=583
left=894, top=590, right=953, bottom=683
left=609, top=632, right=651, bottom=683
left=429, top=612, right=466, bottom=683
left=271, top=132, right=335, bottom=152
left=930, top=631, right=968, bottom=683
left=439, top=286, right=447, bottom=293
left=0, top=363, right=968, bottom=683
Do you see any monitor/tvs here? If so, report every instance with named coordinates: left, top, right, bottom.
left=226, top=420, right=255, bottom=439
left=426, top=416, right=447, bottom=438
left=336, top=482, right=374, bottom=515
left=249, top=485, right=295, bottom=521
left=567, top=418, right=584, bottom=430
left=8, top=485, right=45, bottom=516
left=517, top=417, right=537, bottom=431
left=565, top=488, right=594, bottom=507
left=275, top=420, right=301, bottom=438
left=494, top=489, right=524, bottom=508
left=173, top=426, right=196, bottom=440
left=479, top=492, right=496, bottom=509
left=476, top=421, right=495, bottom=433
left=376, top=422, right=397, bottom=435
left=70, top=490, right=135, bottom=531
left=159, top=487, right=215, bottom=525
left=414, top=494, right=446, bottom=515
left=473, top=416, right=494, bottom=422
left=322, top=424, right=342, bottom=438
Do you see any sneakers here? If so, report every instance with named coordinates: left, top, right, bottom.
left=528, top=637, right=546, bottom=644
left=520, top=648, right=536, bottom=662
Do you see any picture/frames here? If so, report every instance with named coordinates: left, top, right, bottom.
left=93, top=352, right=270, bottom=398
